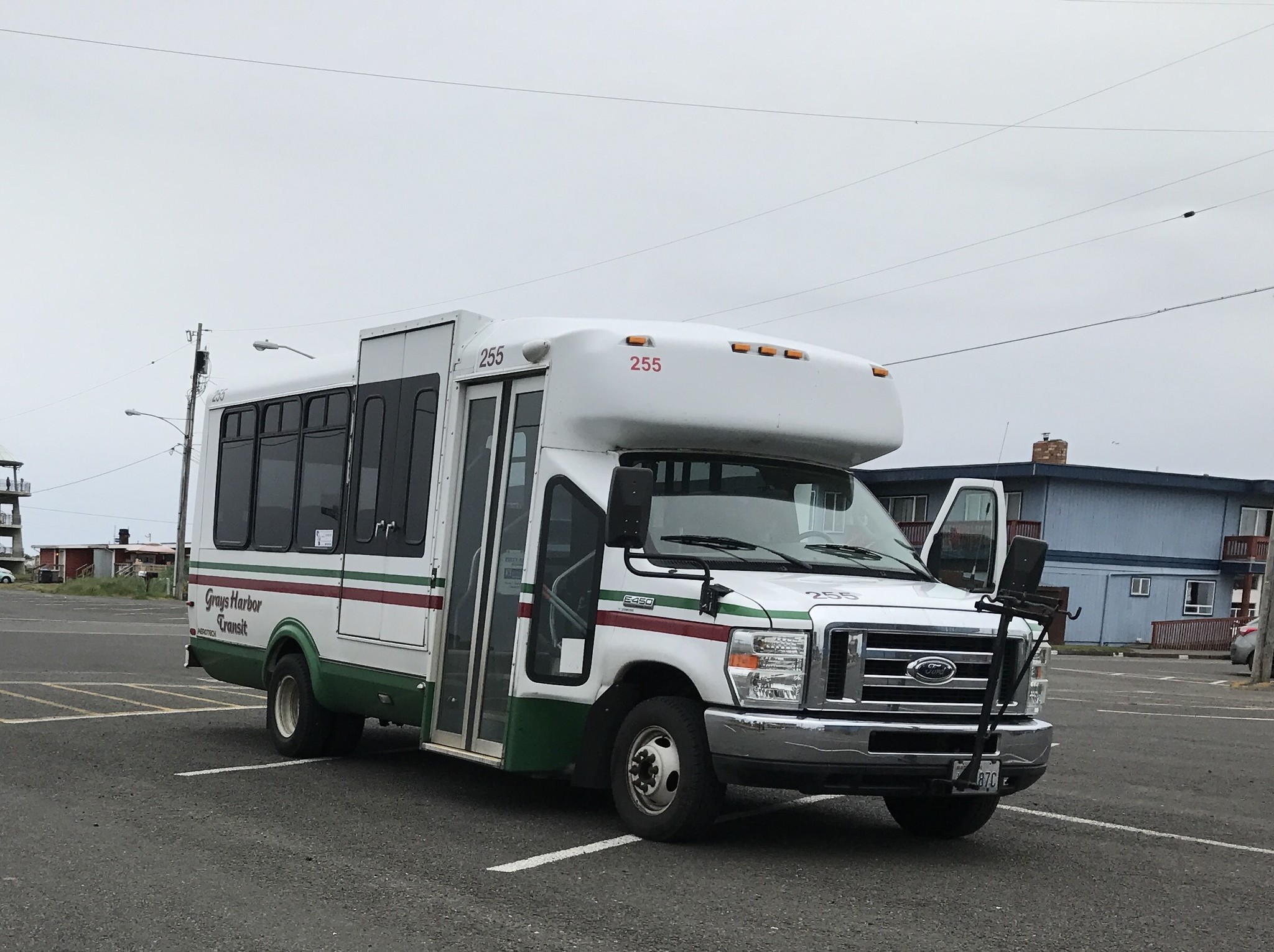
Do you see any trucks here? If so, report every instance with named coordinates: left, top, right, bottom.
left=184, top=308, right=1085, bottom=847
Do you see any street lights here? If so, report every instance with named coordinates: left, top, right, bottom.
left=125, top=408, right=193, bottom=600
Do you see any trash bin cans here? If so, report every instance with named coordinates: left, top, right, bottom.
left=51, top=570, right=60, bottom=583
left=38, top=569, right=51, bottom=583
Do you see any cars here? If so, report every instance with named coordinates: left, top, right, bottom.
left=0, top=567, right=16, bottom=584
left=1230, top=617, right=1274, bottom=679
